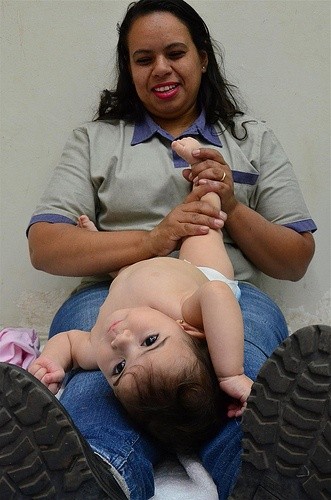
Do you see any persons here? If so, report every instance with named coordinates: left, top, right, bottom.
left=0, top=0, right=331, bottom=500
left=27, top=137, right=255, bottom=449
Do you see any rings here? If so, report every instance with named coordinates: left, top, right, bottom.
left=220, top=172, right=226, bottom=181
